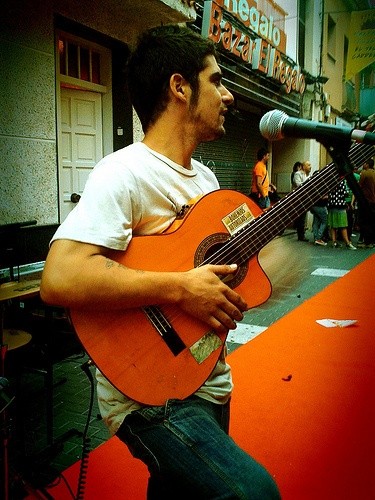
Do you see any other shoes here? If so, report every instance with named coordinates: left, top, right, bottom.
left=298, top=238, right=309, bottom=242
left=357, top=242, right=375, bottom=248
left=314, top=240, right=328, bottom=245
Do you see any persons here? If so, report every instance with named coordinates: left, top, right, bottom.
left=327, top=179, right=357, bottom=250
left=40, top=24, right=281, bottom=500
left=309, top=171, right=328, bottom=246
left=248, top=147, right=277, bottom=212
left=291, top=162, right=310, bottom=242
left=358, top=159, right=375, bottom=247
left=344, top=180, right=355, bottom=243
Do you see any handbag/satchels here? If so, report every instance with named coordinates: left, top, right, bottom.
left=248, top=192, right=259, bottom=204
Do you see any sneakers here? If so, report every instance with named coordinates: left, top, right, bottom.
left=332, top=242, right=342, bottom=248
left=346, top=243, right=357, bottom=250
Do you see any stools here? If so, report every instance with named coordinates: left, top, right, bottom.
left=0, top=330, right=54, bottom=443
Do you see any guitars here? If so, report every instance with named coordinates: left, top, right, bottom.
left=68, top=128, right=375, bottom=405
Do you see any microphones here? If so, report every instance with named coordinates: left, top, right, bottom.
left=258, top=110, right=375, bottom=145
